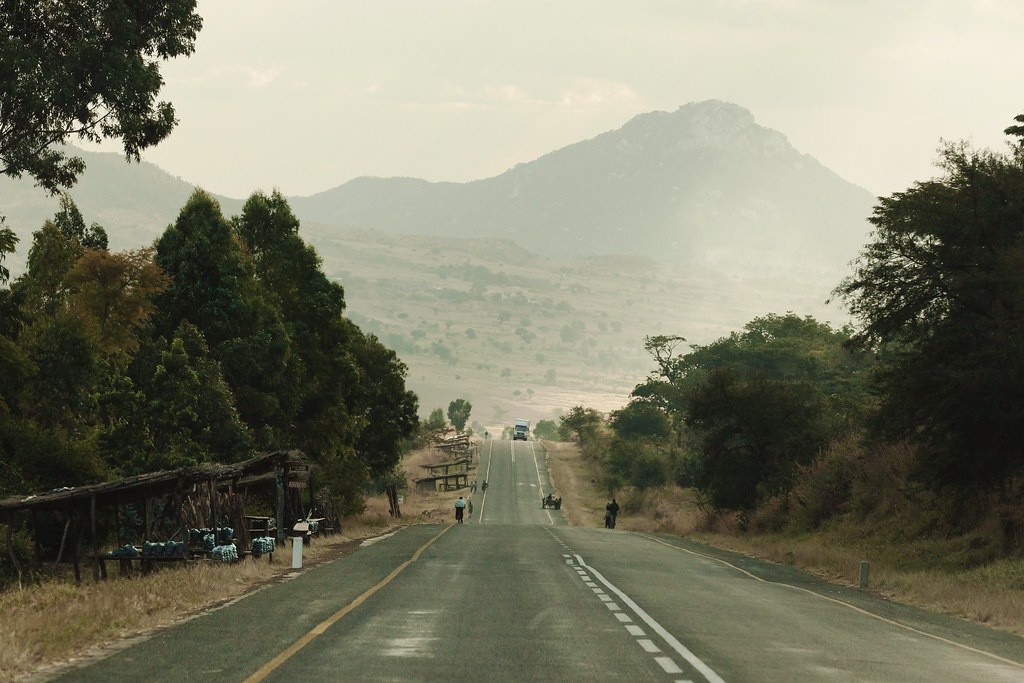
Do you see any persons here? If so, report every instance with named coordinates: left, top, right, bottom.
left=454, top=496, right=466, bottom=524
left=484, top=430, right=488, bottom=442
left=547, top=493, right=562, bottom=509
left=603, top=498, right=620, bottom=529
left=470, top=479, right=488, bottom=494
left=467, top=502, right=473, bottom=517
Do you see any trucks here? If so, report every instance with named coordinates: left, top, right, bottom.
left=512, top=418, right=531, bottom=442
